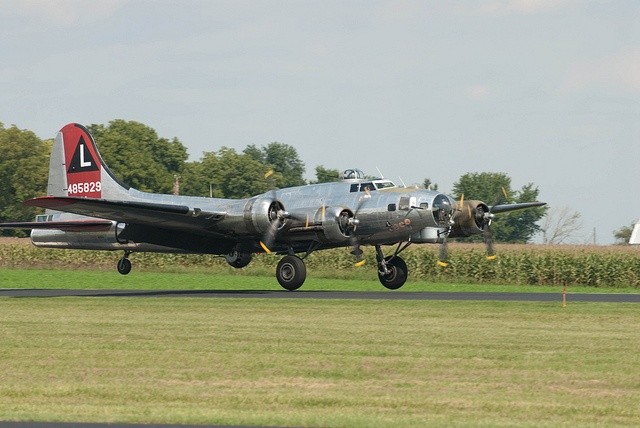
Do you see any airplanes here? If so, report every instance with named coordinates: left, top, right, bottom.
left=0, top=122, right=548, bottom=291
